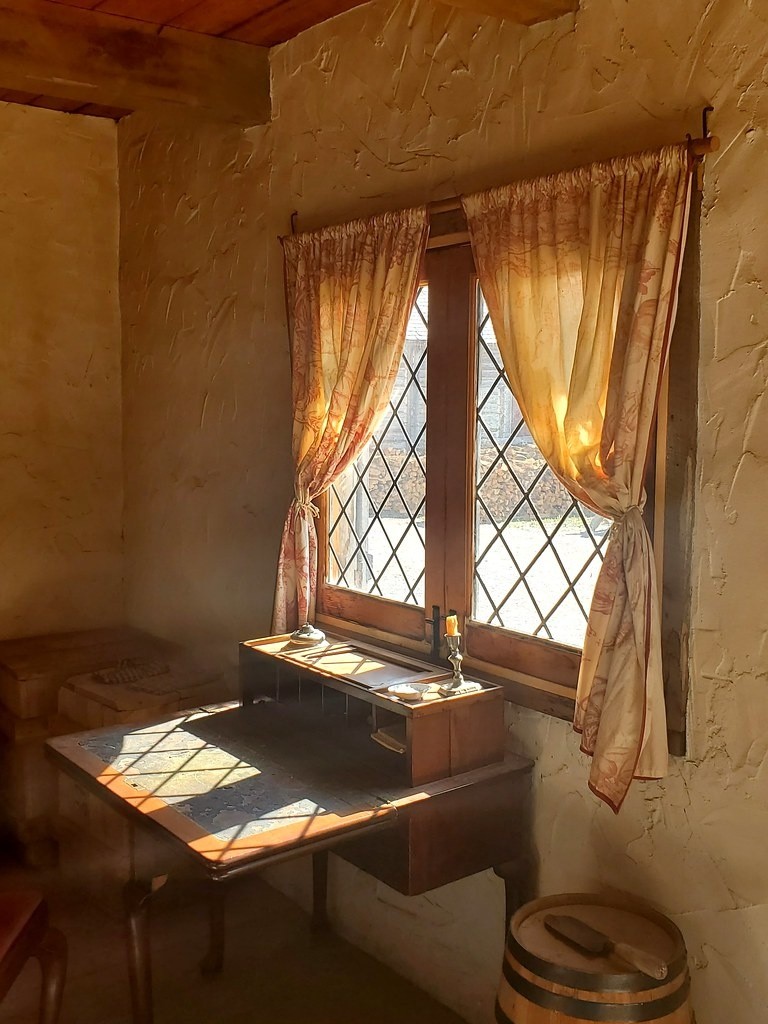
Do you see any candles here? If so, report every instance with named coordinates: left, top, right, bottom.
left=446, top=614, right=459, bottom=636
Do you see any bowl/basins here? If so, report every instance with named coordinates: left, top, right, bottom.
left=388, top=682, right=432, bottom=700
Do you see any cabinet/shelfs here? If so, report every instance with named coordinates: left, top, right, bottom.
left=43, top=630, right=540, bottom=1024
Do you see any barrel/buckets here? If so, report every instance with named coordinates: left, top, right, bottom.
left=494, top=893, right=697, bottom=1024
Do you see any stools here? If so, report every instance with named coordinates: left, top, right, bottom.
left=0, top=890, right=70, bottom=1024
